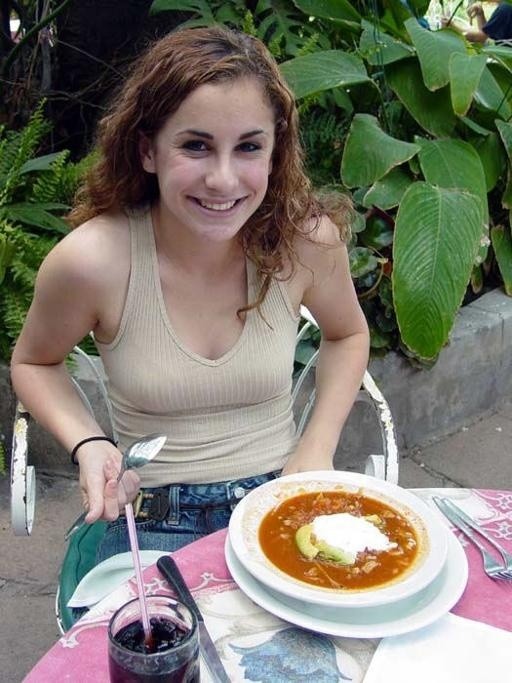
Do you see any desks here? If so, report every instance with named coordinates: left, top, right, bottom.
left=17, top=489, right=511, bottom=683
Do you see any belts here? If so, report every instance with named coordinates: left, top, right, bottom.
left=139, top=473, right=280, bottom=508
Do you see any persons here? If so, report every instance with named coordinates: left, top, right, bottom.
left=7, top=20, right=372, bottom=609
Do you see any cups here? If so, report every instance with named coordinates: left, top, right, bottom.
left=106, top=594, right=203, bottom=683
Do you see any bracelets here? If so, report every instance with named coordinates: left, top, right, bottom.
left=67, top=434, right=119, bottom=467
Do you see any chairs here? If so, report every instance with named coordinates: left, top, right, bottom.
left=9, top=300, right=402, bottom=637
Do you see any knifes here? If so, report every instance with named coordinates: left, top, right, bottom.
left=156, top=555, right=232, bottom=683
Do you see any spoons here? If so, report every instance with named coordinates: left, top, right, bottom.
left=64, top=430, right=168, bottom=541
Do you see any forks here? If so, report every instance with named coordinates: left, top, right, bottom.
left=433, top=495, right=512, bottom=581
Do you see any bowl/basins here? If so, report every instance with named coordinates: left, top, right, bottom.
left=227, top=469, right=451, bottom=610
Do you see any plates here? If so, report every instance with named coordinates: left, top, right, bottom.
left=224, top=527, right=470, bottom=640
